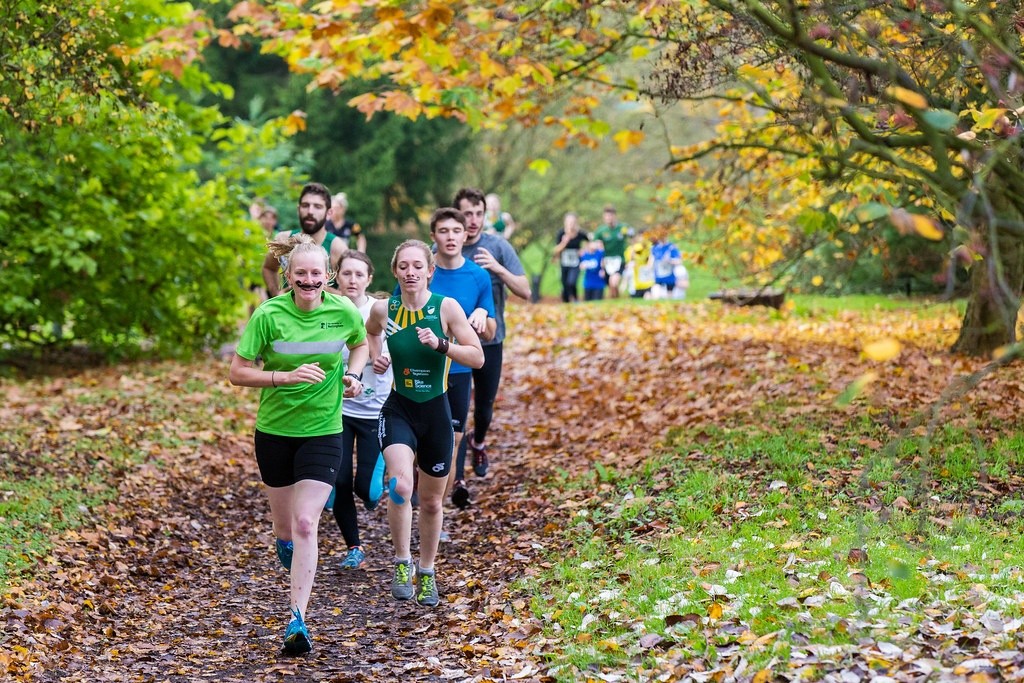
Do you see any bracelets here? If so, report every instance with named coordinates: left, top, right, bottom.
left=436, top=337, right=449, bottom=354
left=271, top=370, right=278, bottom=388
left=345, top=371, right=360, bottom=380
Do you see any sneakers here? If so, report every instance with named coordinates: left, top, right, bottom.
left=275, top=538, right=294, bottom=569
left=338, top=547, right=366, bottom=575
left=467, top=429, right=489, bottom=477
left=391, top=555, right=416, bottom=600
left=281, top=604, right=312, bottom=656
left=363, top=499, right=378, bottom=509
left=451, top=480, right=471, bottom=511
left=415, top=559, right=440, bottom=609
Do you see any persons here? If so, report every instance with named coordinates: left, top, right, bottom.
left=229, top=233, right=369, bottom=655
left=258, top=180, right=368, bottom=298
left=332, top=248, right=393, bottom=571
left=553, top=205, right=634, bottom=303
left=428, top=185, right=533, bottom=477
left=484, top=194, right=516, bottom=238
left=391, top=207, right=496, bottom=545
left=363, top=239, right=485, bottom=608
left=624, top=225, right=689, bottom=301
